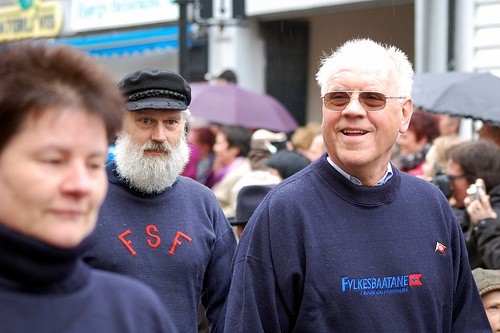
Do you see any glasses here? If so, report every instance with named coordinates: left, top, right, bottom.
left=448, top=174, right=465, bottom=181
left=321, top=91, right=409, bottom=111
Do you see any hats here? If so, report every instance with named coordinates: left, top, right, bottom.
left=226, top=184, right=276, bottom=225
left=471, top=267, right=500, bottom=295
left=116, top=67, right=192, bottom=111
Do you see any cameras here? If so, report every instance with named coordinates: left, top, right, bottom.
left=466, top=179, right=486, bottom=201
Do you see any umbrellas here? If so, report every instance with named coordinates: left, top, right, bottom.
left=187, top=81, right=299, bottom=133
left=409, top=69, right=500, bottom=122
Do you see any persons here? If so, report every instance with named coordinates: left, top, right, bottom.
left=226, top=38, right=491, bottom=333
left=183, top=121, right=326, bottom=241
left=390, top=107, right=500, bottom=333
left=0, top=43, right=178, bottom=333
left=79, top=66, right=238, bottom=333
